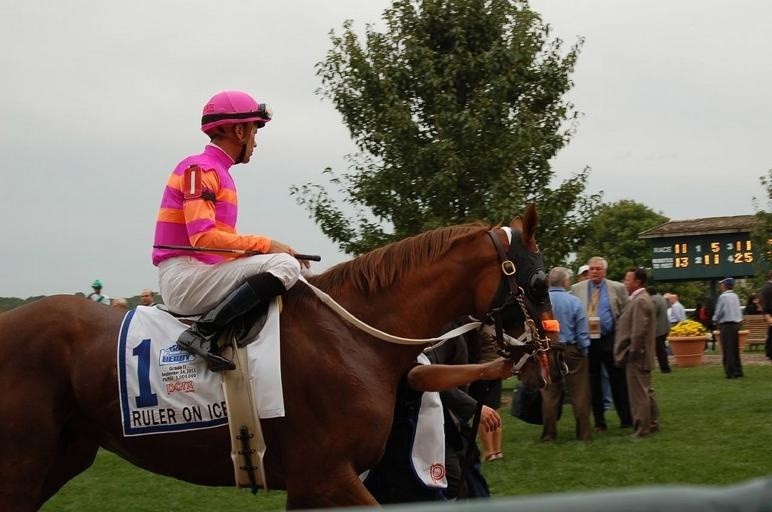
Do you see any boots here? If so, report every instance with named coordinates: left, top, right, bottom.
left=175, top=281, right=264, bottom=371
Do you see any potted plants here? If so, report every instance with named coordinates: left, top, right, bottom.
left=712, top=325, right=749, bottom=354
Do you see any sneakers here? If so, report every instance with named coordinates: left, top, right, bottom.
left=495, top=451, right=504, bottom=459
left=480, top=452, right=494, bottom=462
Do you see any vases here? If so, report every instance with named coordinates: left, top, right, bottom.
left=667, top=336, right=708, bottom=366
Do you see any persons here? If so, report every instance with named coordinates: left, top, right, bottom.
left=368, top=336, right=512, bottom=505
left=646, top=285, right=672, bottom=374
left=759, top=271, right=772, bottom=358
left=662, top=292, right=678, bottom=327
left=537, top=267, right=601, bottom=445
left=691, top=297, right=716, bottom=350
left=474, top=332, right=504, bottom=464
left=571, top=264, right=591, bottom=286
left=613, top=267, right=661, bottom=439
left=743, top=295, right=764, bottom=315
left=151, top=91, right=302, bottom=371
left=712, top=277, right=744, bottom=379
left=112, top=297, right=127, bottom=312
left=138, top=291, right=156, bottom=308
left=669, top=294, right=687, bottom=328
left=85, top=280, right=110, bottom=306
left=570, top=256, right=633, bottom=434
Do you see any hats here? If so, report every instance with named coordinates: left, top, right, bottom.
left=576, top=264, right=589, bottom=277
left=718, top=278, right=735, bottom=285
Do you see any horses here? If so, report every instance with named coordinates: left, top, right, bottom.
left=0, top=204, right=559, bottom=512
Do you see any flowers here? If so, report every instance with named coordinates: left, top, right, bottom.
left=669, top=320, right=706, bottom=337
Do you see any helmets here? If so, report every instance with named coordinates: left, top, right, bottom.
left=201, top=90, right=272, bottom=132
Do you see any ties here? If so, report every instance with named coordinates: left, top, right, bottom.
left=588, top=287, right=600, bottom=316
